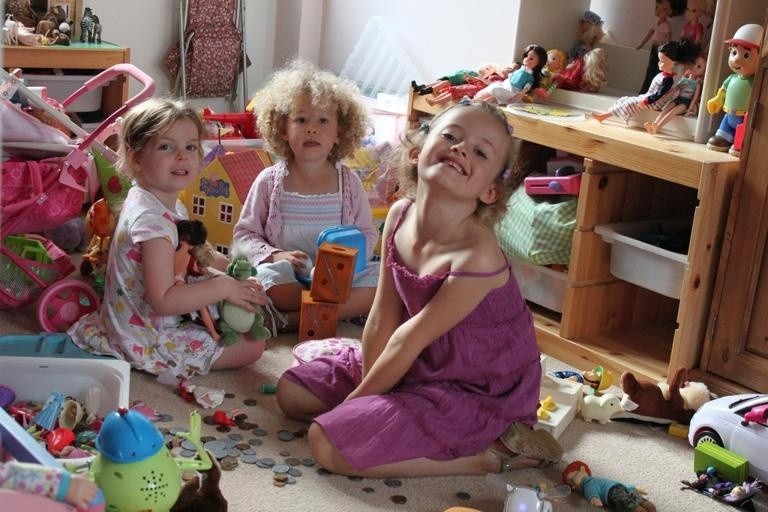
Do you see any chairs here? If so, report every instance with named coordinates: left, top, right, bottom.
left=165, top=0, right=252, bottom=116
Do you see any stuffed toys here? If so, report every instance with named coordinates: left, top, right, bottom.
left=0, top=0, right=104, bottom=46
left=1, top=60, right=276, bottom=346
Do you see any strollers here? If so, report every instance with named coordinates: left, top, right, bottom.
left=0, top=56, right=162, bottom=336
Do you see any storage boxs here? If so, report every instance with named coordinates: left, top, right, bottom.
left=504, top=252, right=567, bottom=313
left=1, top=356, right=130, bottom=479
left=589, top=215, right=691, bottom=299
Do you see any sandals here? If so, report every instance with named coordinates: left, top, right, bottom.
left=485, top=421, right=564, bottom=473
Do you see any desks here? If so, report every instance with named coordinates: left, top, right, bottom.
left=0, top=36, right=132, bottom=155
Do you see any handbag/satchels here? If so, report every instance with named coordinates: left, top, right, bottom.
left=0, top=156, right=89, bottom=236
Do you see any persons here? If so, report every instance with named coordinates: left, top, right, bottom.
left=276, top=93, right=565, bottom=473
left=409, top=0, right=712, bottom=194
left=1, top=374, right=250, bottom=512
left=592, top=0, right=713, bottom=134
left=224, top=64, right=382, bottom=335
left=410, top=44, right=607, bottom=107
left=704, top=21, right=765, bottom=158
left=533, top=356, right=766, bottom=512
left=570, top=12, right=604, bottom=60
left=65, top=95, right=266, bottom=375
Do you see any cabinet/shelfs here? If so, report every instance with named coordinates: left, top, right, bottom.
left=687, top=39, right=768, bottom=418
left=404, top=86, right=739, bottom=396
